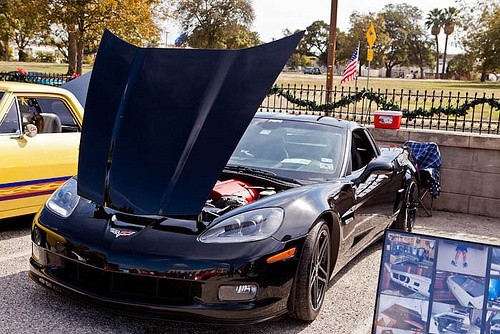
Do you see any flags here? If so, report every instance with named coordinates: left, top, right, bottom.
left=340, top=42, right=359, bottom=86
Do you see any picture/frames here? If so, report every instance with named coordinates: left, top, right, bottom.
left=371, top=228, right=500, bottom=334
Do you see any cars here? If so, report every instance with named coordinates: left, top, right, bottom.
left=0, top=71, right=93, bottom=219
left=433, top=307, right=470, bottom=333
left=303, top=66, right=322, bottom=74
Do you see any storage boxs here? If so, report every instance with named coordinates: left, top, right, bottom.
left=373, top=110, right=403, bottom=128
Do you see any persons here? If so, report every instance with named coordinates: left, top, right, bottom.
left=451, top=244, right=469, bottom=268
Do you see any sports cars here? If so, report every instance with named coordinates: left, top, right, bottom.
left=27, top=27, right=423, bottom=326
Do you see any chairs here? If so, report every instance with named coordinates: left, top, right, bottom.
left=39, top=112, right=62, bottom=134
left=402, top=139, right=443, bottom=218
left=323, top=133, right=363, bottom=173
left=248, top=131, right=289, bottom=161
